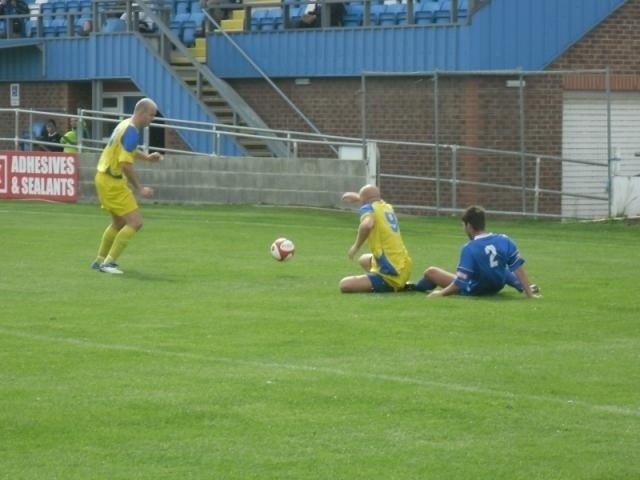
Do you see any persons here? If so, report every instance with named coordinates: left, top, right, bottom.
left=35, top=118, right=64, bottom=152
left=91, top=98, right=163, bottom=273
left=0, top=0, right=30, bottom=37
left=121, top=0, right=158, bottom=33
left=337, top=184, right=420, bottom=292
left=59, top=118, right=91, bottom=153
left=300, top=3, right=346, bottom=27
left=412, top=204, right=541, bottom=300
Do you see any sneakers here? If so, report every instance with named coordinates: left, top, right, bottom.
left=405, top=283, right=416, bottom=290
left=529, top=284, right=538, bottom=292
left=92, top=262, right=122, bottom=274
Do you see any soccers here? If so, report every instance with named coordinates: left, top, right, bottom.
left=270, top=237, right=295, bottom=262
